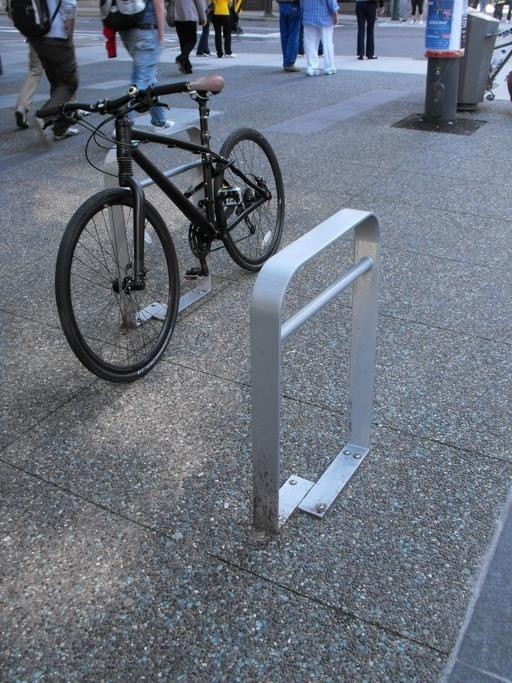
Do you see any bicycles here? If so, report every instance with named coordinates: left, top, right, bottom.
left=461, top=28, right=512, bottom=101
left=35, top=76, right=285, bottom=382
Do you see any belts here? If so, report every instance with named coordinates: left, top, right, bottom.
left=139, top=24, right=157, bottom=30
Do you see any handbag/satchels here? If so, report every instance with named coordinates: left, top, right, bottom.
left=167, top=1, right=174, bottom=27
left=206, top=3, right=214, bottom=19
left=8, top=0, right=50, bottom=38
left=100, top=0, right=148, bottom=30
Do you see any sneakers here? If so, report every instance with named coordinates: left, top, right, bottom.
left=307, top=70, right=320, bottom=77
left=358, top=56, right=378, bottom=60
left=32, top=116, right=51, bottom=146
left=78, top=109, right=91, bottom=116
left=54, top=128, right=78, bottom=141
left=197, top=52, right=236, bottom=58
left=285, top=66, right=299, bottom=72
left=325, top=69, right=336, bottom=75
left=152, top=121, right=175, bottom=131
left=15, top=106, right=30, bottom=129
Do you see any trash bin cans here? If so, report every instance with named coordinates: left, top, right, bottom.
left=457, top=7, right=502, bottom=111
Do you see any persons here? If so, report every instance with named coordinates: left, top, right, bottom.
left=15, top=41, right=91, bottom=129
left=164, top=0, right=248, bottom=74
left=355, top=0, right=385, bottom=60
left=411, top=0, right=425, bottom=24
left=112, top=0, right=175, bottom=139
left=276, top=0, right=340, bottom=76
left=468, top=0, right=512, bottom=23
left=27, top=0, right=80, bottom=141
